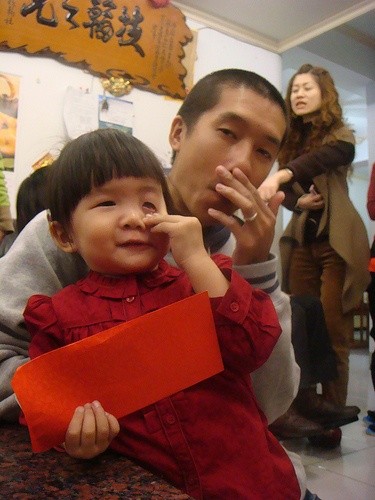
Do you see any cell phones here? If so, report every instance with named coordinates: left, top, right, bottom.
left=309, top=185, right=319, bottom=196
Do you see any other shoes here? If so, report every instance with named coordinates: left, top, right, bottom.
left=367, top=410, right=375, bottom=420
left=294, top=390, right=362, bottom=427
left=367, top=423, right=375, bottom=436
